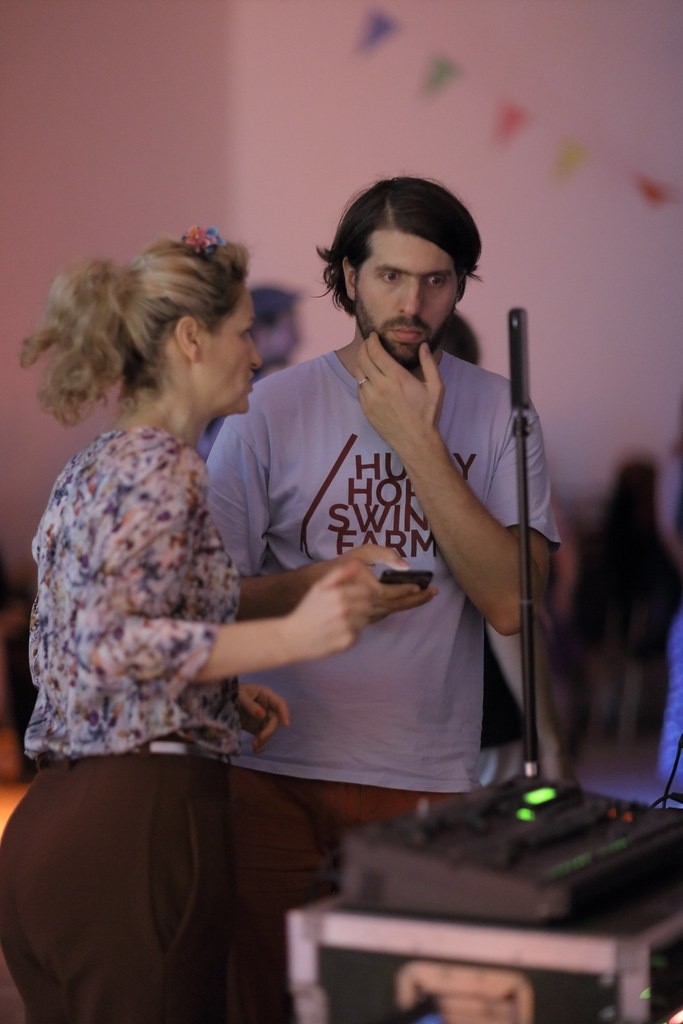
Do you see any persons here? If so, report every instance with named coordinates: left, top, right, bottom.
left=205, top=178, right=563, bottom=1024
left=0, top=225, right=371, bottom=1023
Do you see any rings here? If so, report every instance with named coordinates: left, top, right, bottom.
left=358, top=377, right=368, bottom=388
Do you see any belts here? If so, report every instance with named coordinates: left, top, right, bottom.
left=149, top=741, right=188, bottom=754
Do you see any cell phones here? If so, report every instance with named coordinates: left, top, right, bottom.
left=380, top=570, right=433, bottom=594
